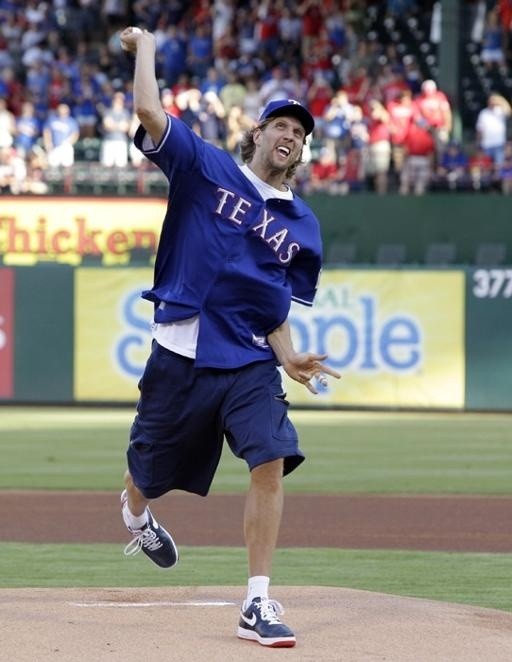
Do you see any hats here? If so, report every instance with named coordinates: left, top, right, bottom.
left=258, top=97, right=315, bottom=136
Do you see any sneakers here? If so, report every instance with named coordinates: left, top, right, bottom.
left=121, top=488, right=179, bottom=568
left=236, top=597, right=296, bottom=648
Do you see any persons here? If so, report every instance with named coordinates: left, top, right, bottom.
left=120, top=22, right=340, bottom=647
left=1, top=0, right=512, bottom=195
